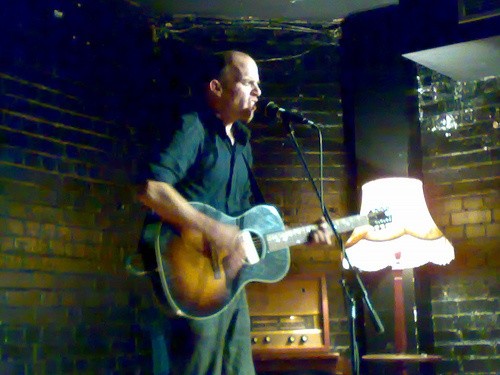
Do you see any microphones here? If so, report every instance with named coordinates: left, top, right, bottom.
left=258, top=97, right=320, bottom=131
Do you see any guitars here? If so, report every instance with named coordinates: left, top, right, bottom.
left=140, top=200, right=392, bottom=321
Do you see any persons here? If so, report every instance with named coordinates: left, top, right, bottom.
left=134, top=47, right=337, bottom=375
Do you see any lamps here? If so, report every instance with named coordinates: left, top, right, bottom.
left=341, top=176, right=457, bottom=363
